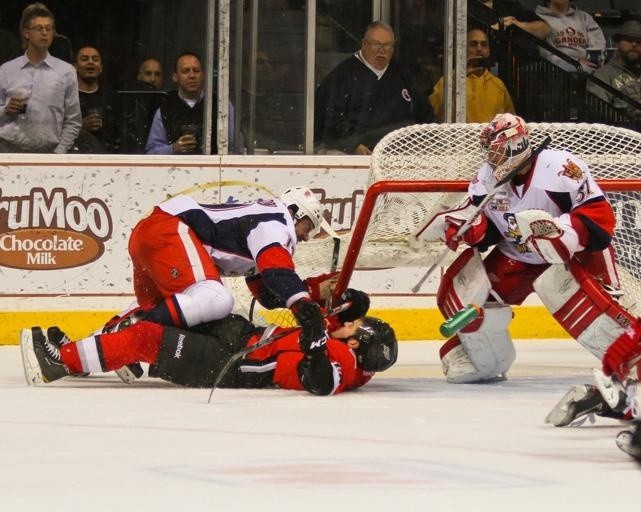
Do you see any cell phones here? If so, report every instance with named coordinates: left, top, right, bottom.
left=468, top=57, right=495, bottom=69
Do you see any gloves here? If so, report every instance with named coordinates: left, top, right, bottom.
left=305, top=271, right=342, bottom=304
left=339, top=288, right=369, bottom=321
left=292, top=300, right=327, bottom=352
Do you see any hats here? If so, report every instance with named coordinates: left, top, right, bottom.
left=613, top=21, right=641, bottom=39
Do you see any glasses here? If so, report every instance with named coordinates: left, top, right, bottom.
left=26, top=25, right=54, bottom=33
left=365, top=39, right=394, bottom=52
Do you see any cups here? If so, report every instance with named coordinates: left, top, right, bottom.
left=586, top=48, right=601, bottom=69
left=19, top=104, right=27, bottom=114
left=181, top=125, right=198, bottom=137
left=603, top=48, right=619, bottom=61
left=88, top=108, right=99, bottom=130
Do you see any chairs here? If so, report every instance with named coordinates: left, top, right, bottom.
left=467, top=0, right=641, bottom=81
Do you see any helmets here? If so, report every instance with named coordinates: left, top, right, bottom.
left=480, top=113, right=532, bottom=181
left=346, top=317, right=398, bottom=371
left=281, top=187, right=323, bottom=229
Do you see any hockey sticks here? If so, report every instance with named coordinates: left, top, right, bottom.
left=319, top=218, right=341, bottom=275
left=209, top=299, right=353, bottom=401
left=411, top=137, right=554, bottom=295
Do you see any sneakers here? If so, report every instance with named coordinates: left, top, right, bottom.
left=103, top=315, right=143, bottom=378
left=31, top=327, right=69, bottom=383
left=556, top=383, right=601, bottom=427
left=47, top=326, right=71, bottom=347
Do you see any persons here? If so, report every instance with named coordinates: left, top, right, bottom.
left=535, top=372, right=640, bottom=460
left=413, top=113, right=641, bottom=465
left=20, top=290, right=397, bottom=395
left=396, top=0, right=640, bottom=132
left=88, top=186, right=324, bottom=384
left=72, top=43, right=245, bottom=155
left=0, top=4, right=83, bottom=154
left=314, top=20, right=438, bottom=155
